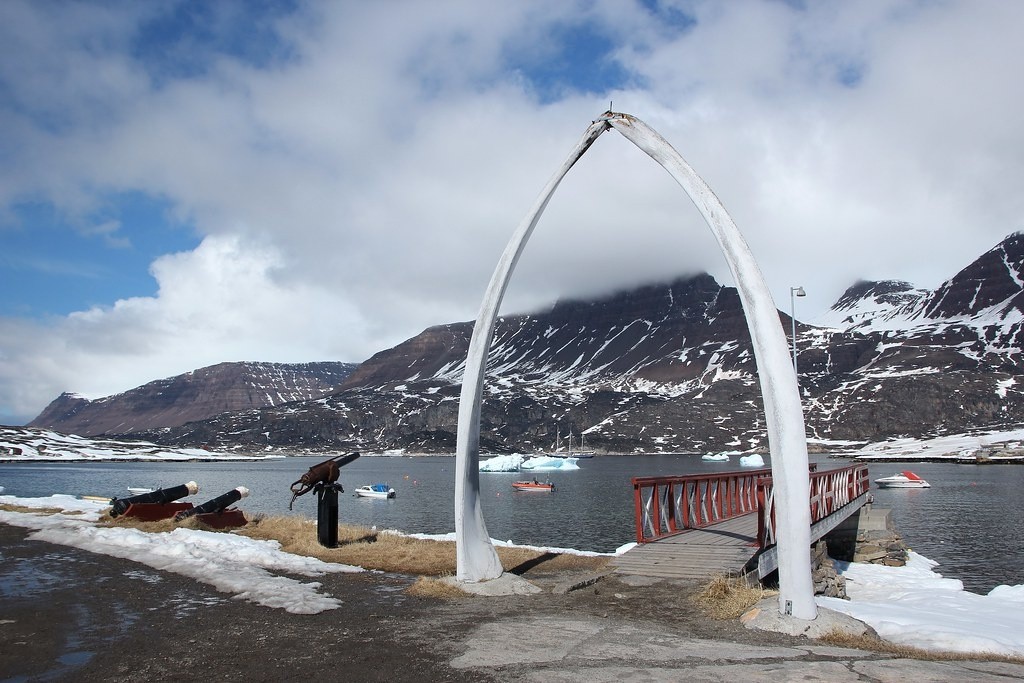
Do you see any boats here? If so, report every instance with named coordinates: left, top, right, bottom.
left=875, top=471, right=931, bottom=488
left=355, top=485, right=396, bottom=499
left=702, top=451, right=729, bottom=462
left=513, top=479, right=555, bottom=493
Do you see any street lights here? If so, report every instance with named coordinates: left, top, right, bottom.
left=790, top=286, right=806, bottom=372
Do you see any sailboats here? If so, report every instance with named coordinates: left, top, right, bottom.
left=545, top=426, right=598, bottom=459
